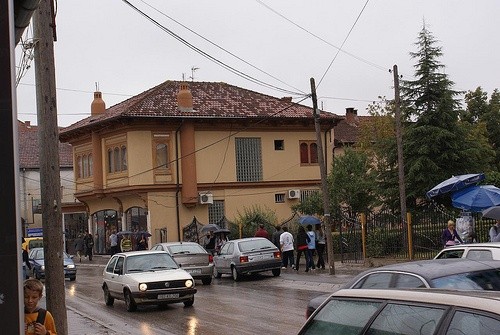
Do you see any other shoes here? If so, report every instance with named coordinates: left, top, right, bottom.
left=281, top=267, right=287, bottom=270
left=305, top=266, right=325, bottom=272
left=293, top=267, right=298, bottom=270
left=292, top=264, right=295, bottom=269
left=89, top=258, right=92, bottom=261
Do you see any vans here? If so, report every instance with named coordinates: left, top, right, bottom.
left=22, top=237, right=43, bottom=253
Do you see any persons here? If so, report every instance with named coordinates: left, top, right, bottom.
left=489, top=220, right=500, bottom=242
left=254, top=224, right=269, bottom=239
left=272, top=226, right=282, bottom=248
left=279, top=226, right=295, bottom=269
left=293, top=226, right=311, bottom=272
left=203, top=231, right=229, bottom=255
left=305, top=225, right=316, bottom=270
left=108, top=231, right=148, bottom=257
left=23, top=279, right=57, bottom=335
left=74, top=233, right=94, bottom=262
left=22, top=249, right=32, bottom=279
left=314, top=223, right=327, bottom=270
left=441, top=220, right=464, bottom=249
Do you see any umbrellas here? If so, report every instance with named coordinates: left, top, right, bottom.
left=135, top=231, right=152, bottom=237
left=300, top=216, right=320, bottom=225
left=201, top=224, right=231, bottom=236
left=116, top=230, right=133, bottom=236
left=482, top=206, right=500, bottom=220
left=426, top=173, right=486, bottom=200
left=451, top=184, right=500, bottom=212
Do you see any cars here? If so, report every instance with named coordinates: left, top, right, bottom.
left=306, top=257, right=500, bottom=320
left=212, top=237, right=282, bottom=282
left=433, top=242, right=500, bottom=260
left=150, top=241, right=215, bottom=285
left=26, top=247, right=78, bottom=281
left=102, top=250, right=197, bottom=312
left=297, top=288, right=500, bottom=335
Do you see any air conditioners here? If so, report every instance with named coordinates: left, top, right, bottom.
left=288, top=189, right=300, bottom=199
left=199, top=193, right=213, bottom=204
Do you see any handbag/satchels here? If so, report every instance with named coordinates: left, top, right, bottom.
left=296, top=244, right=308, bottom=252
